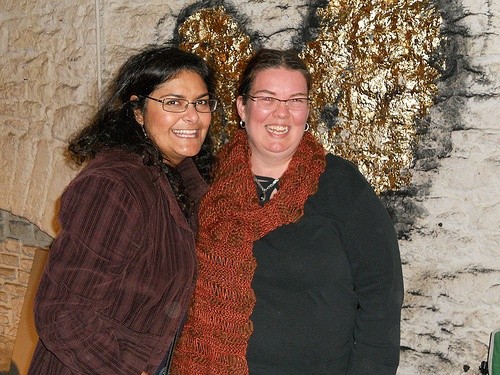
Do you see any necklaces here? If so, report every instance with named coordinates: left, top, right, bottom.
left=251, top=174, right=279, bottom=201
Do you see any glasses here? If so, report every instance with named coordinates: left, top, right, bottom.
left=138, top=92, right=218, bottom=113
left=242, top=93, right=310, bottom=111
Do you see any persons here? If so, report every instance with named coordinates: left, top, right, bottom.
left=164, top=47, right=404, bottom=375
left=27, top=44, right=220, bottom=375
